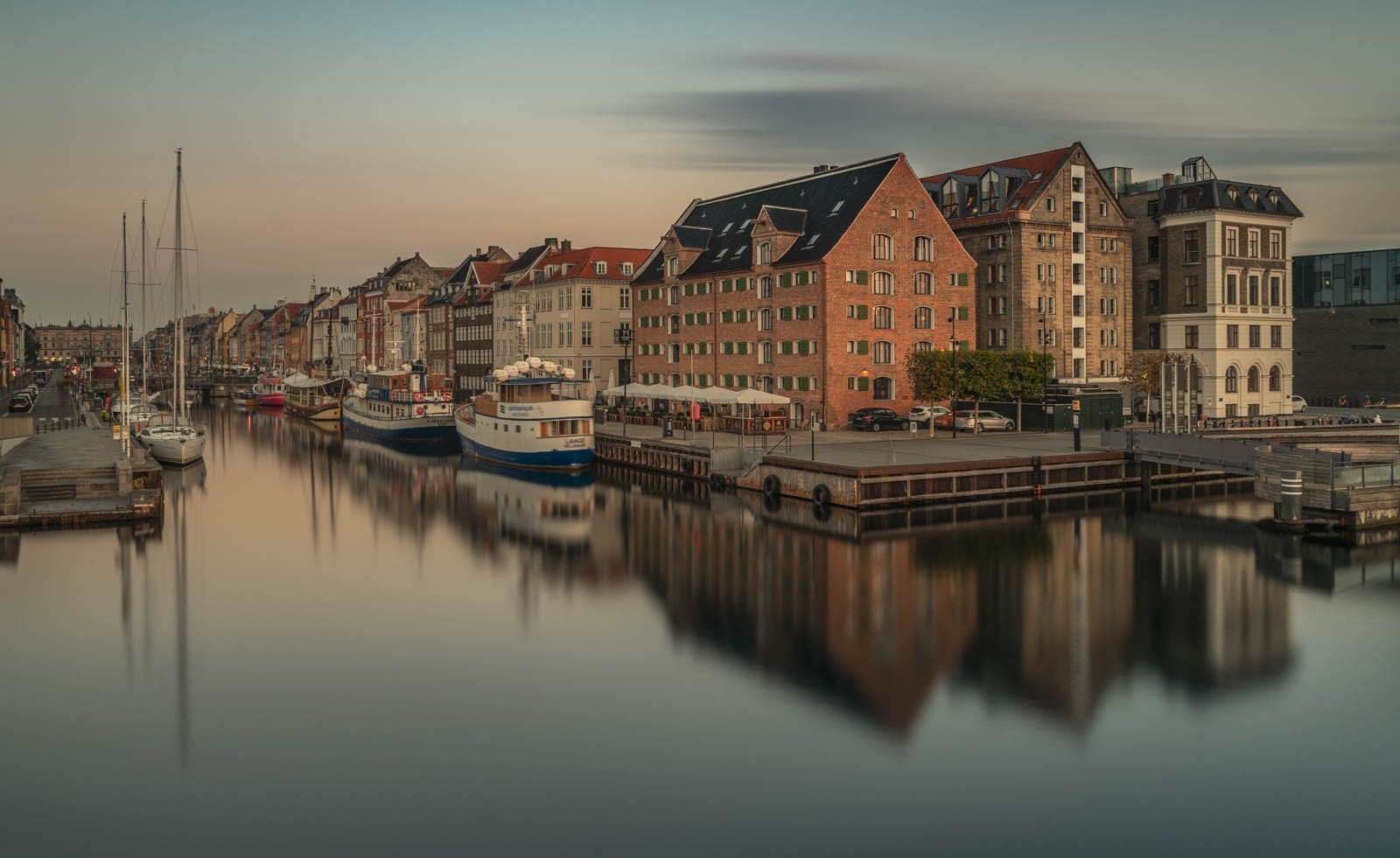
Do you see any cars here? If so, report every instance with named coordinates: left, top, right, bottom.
left=9, top=385, right=39, bottom=411
left=908, top=406, right=953, bottom=429
left=955, top=411, right=1015, bottom=433
left=1292, top=396, right=1308, bottom=413
left=851, top=408, right=910, bottom=432
left=934, top=412, right=955, bottom=430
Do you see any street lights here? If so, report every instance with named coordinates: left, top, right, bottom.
left=1039, top=318, right=1048, bottom=432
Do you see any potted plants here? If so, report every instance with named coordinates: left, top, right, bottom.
left=607, top=404, right=686, bottom=429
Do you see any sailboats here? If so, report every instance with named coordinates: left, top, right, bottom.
left=282, top=281, right=356, bottom=421
left=106, top=147, right=292, bottom=466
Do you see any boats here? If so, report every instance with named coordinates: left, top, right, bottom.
left=342, top=294, right=456, bottom=440
left=453, top=289, right=597, bottom=467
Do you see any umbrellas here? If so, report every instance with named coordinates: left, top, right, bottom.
left=586, top=369, right=596, bottom=404
left=607, top=369, right=616, bottom=408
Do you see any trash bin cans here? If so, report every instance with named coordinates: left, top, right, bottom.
left=88, top=390, right=94, bottom=400
left=594, top=409, right=608, bottom=424
left=1280, top=469, right=1303, bottom=521
left=94, top=398, right=102, bottom=410
left=1294, top=419, right=1308, bottom=426
left=662, top=415, right=674, bottom=438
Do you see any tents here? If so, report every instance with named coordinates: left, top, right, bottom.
left=603, top=382, right=791, bottom=435
